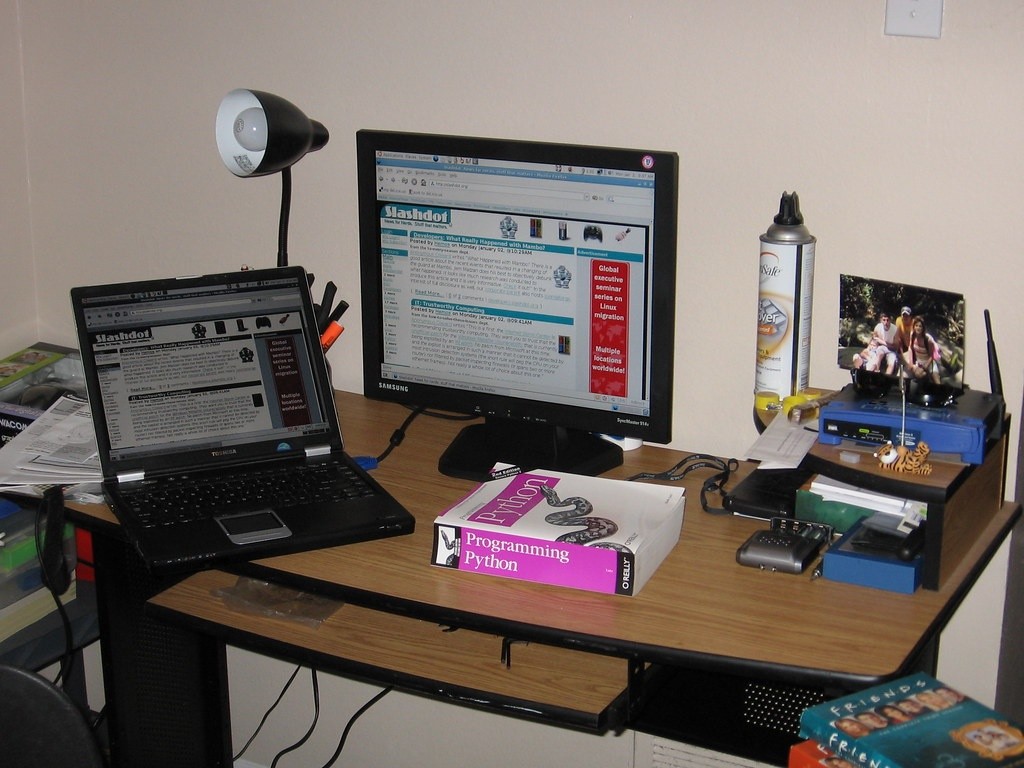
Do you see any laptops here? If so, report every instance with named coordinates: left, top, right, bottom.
left=68, top=266, right=417, bottom=574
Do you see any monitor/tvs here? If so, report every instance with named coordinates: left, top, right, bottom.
left=355, top=128, right=681, bottom=482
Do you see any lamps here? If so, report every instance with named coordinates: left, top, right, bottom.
left=214, top=89, right=330, bottom=267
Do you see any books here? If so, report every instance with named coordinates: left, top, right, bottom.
left=788, top=739, right=858, bottom=768
left=430, top=462, right=686, bottom=598
left=800, top=672, right=1024, bottom=768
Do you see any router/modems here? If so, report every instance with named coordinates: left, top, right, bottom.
left=815, top=309, right=1009, bottom=465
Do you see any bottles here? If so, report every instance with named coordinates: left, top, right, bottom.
left=755, top=191, right=817, bottom=402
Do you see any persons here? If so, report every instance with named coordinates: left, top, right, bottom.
left=974, top=728, right=1016, bottom=750
left=853, top=306, right=941, bottom=384
left=825, top=757, right=853, bottom=768
left=836, top=688, right=970, bottom=738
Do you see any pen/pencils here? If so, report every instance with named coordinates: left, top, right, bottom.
left=318, top=280, right=338, bottom=333
left=319, top=320, right=345, bottom=355
left=306, top=272, right=315, bottom=289
left=321, top=300, right=350, bottom=335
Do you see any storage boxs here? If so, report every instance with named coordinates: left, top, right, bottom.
left=0, top=340, right=89, bottom=447
left=822, top=515, right=923, bottom=594
left=0, top=388, right=1024, bottom=768
left=0, top=498, right=77, bottom=610
